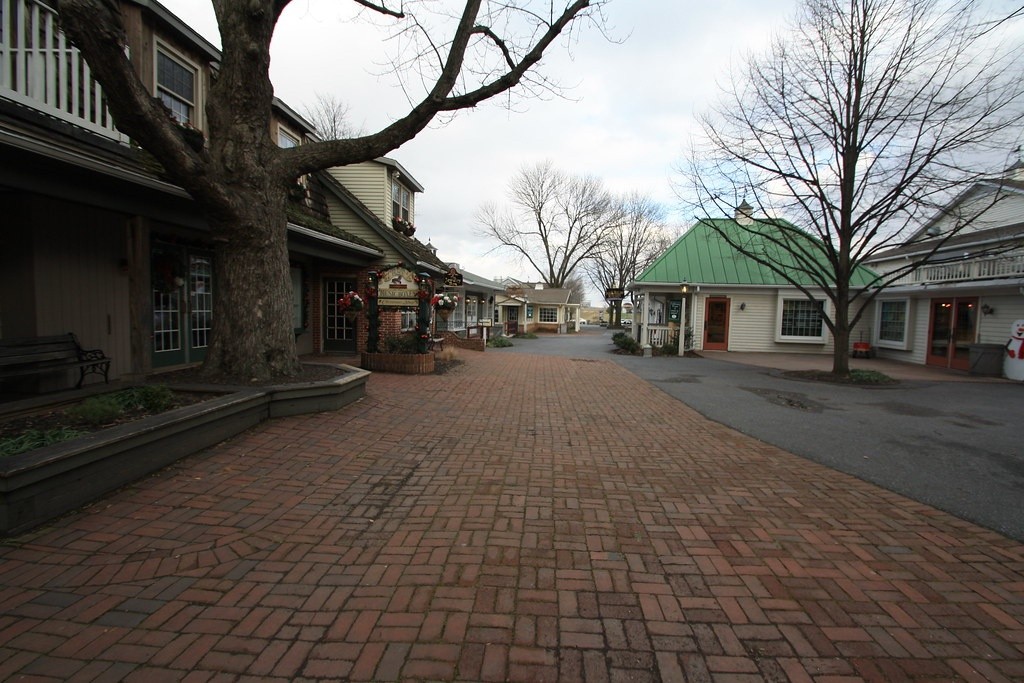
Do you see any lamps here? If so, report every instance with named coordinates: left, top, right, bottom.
left=465, top=297, right=471, bottom=304
left=737, top=302, right=746, bottom=312
left=981, top=304, right=993, bottom=316
left=393, top=171, right=400, bottom=179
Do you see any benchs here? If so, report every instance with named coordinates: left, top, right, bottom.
left=0, top=332, right=113, bottom=404
left=427, top=333, right=445, bottom=351
